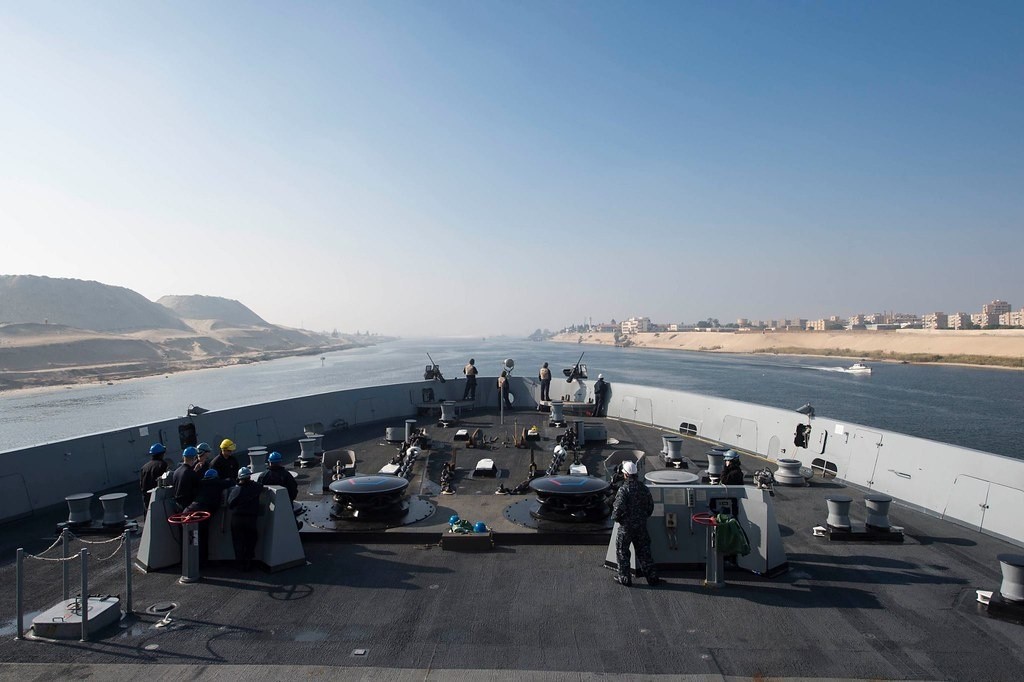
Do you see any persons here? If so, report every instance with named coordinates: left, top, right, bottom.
left=609, top=462, right=666, bottom=586
left=496, top=371, right=514, bottom=410
left=720, top=449, right=745, bottom=516
left=258, top=452, right=303, bottom=531
left=592, top=374, right=607, bottom=417
left=463, top=359, right=478, bottom=400
left=538, top=362, right=553, bottom=401
left=171, top=438, right=238, bottom=572
left=139, top=443, right=170, bottom=522
left=227, top=466, right=263, bottom=569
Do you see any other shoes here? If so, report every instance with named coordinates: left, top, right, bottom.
left=613, top=575, right=632, bottom=587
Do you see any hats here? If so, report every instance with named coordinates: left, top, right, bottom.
left=723, top=451, right=739, bottom=462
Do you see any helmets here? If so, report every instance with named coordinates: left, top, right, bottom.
left=268, top=452, right=282, bottom=463
left=148, top=443, right=167, bottom=454
left=237, top=467, right=251, bottom=479
left=183, top=442, right=212, bottom=458
left=220, top=439, right=237, bottom=451
left=448, top=514, right=461, bottom=527
left=205, top=469, right=217, bottom=481
left=598, top=374, right=604, bottom=378
left=621, top=462, right=637, bottom=475
left=474, top=522, right=486, bottom=532
